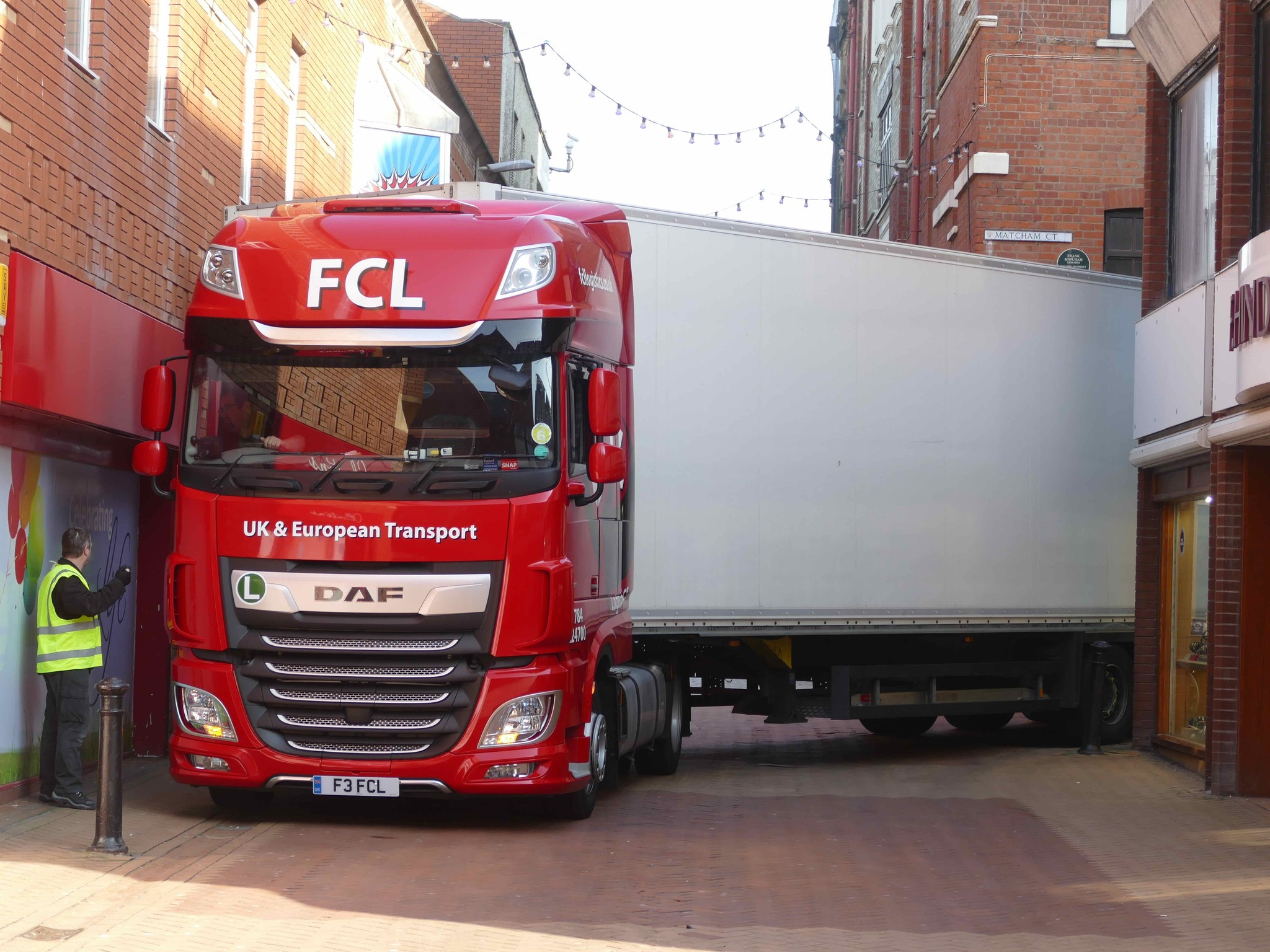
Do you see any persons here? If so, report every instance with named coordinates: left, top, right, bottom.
left=229, top=388, right=307, bottom=452
left=36, top=527, right=131, bottom=810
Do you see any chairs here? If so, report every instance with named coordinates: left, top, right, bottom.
left=417, top=412, right=478, bottom=454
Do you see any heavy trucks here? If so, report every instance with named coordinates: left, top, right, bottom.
left=133, top=181, right=1145, bottom=823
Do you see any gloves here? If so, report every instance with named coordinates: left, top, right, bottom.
left=114, top=564, right=131, bottom=586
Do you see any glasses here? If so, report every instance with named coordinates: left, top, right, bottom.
left=218, top=402, right=239, bottom=416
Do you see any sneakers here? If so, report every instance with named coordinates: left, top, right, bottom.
left=50, top=791, right=96, bottom=810
left=39, top=788, right=53, bottom=802
